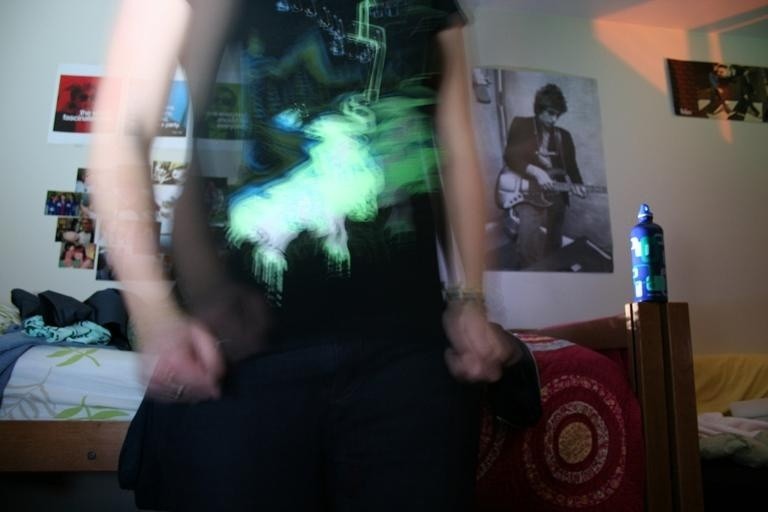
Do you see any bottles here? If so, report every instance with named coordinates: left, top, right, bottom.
left=630, top=203, right=668, bottom=305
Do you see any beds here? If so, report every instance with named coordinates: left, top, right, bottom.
left=665, top=301, right=768, bottom=511
left=0, top=298, right=675, bottom=510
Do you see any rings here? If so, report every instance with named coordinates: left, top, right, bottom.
left=169, top=383, right=187, bottom=401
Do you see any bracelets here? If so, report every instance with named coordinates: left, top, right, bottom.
left=443, top=284, right=484, bottom=303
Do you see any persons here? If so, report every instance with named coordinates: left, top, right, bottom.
left=689, top=63, right=754, bottom=120
left=501, top=83, right=587, bottom=269
left=82, top=0, right=509, bottom=512
left=50, top=167, right=120, bottom=281
left=53, top=82, right=97, bottom=134
